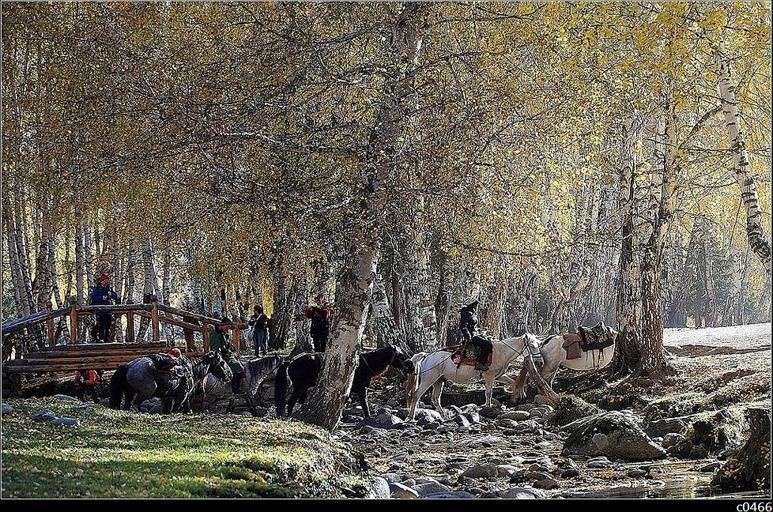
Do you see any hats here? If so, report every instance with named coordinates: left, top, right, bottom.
left=100, top=273, right=109, bottom=282
left=220, top=317, right=232, bottom=325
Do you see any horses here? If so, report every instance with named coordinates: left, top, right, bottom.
left=108, top=344, right=245, bottom=414
left=403, top=331, right=545, bottom=423
left=274, top=335, right=415, bottom=418
left=511, top=325, right=620, bottom=402
left=162, top=351, right=293, bottom=416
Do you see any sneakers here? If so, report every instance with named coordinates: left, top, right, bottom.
left=232, top=389, right=246, bottom=394
left=473, top=364, right=489, bottom=371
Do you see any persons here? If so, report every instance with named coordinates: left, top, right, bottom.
left=207, top=316, right=245, bottom=397
left=304, top=293, right=331, bottom=353
left=248, top=303, right=269, bottom=357
left=457, top=301, right=492, bottom=373
left=73, top=369, right=100, bottom=403
left=212, top=311, right=220, bottom=323
left=91, top=274, right=118, bottom=344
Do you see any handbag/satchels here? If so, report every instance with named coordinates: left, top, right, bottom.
left=243, top=328, right=254, bottom=342
